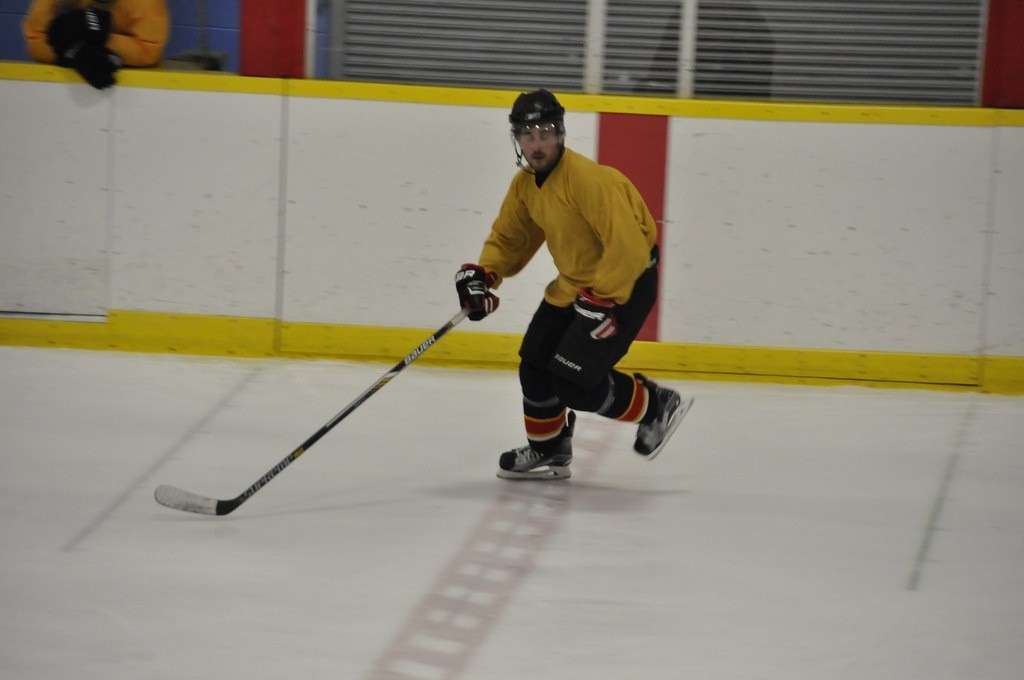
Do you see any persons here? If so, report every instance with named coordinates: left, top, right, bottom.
left=23, top=0, right=169, bottom=89
left=455, top=88, right=680, bottom=473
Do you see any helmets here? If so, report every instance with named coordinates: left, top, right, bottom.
left=509, top=88, right=564, bottom=130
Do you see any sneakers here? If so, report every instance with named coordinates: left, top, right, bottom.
left=497, top=411, right=576, bottom=478
left=633, top=372, right=694, bottom=459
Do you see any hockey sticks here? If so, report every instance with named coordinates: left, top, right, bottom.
left=153, top=306, right=471, bottom=516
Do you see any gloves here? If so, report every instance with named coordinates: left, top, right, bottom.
left=74, top=46, right=119, bottom=89
left=573, top=285, right=618, bottom=338
left=44, top=9, right=111, bottom=46
left=455, top=264, right=500, bottom=321
